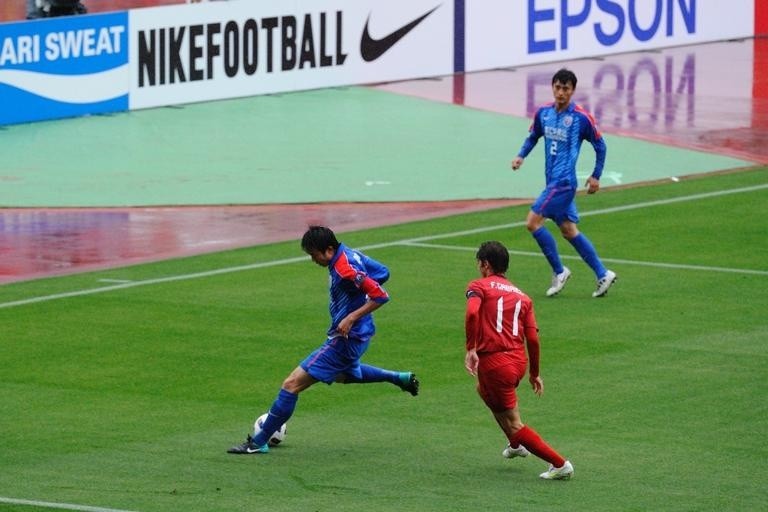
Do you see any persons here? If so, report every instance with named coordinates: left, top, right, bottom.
left=462, top=240, right=575, bottom=482
left=510, top=67, right=621, bottom=301
left=27, top=0, right=84, bottom=22
left=225, top=225, right=422, bottom=456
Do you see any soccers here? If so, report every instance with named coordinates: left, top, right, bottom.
left=254, top=414, right=286, bottom=446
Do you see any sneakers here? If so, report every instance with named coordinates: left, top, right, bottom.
left=544, top=264, right=574, bottom=298
left=398, top=371, right=420, bottom=398
left=501, top=444, right=531, bottom=459
left=539, top=458, right=576, bottom=482
left=591, top=268, right=617, bottom=298
left=225, top=432, right=270, bottom=455
